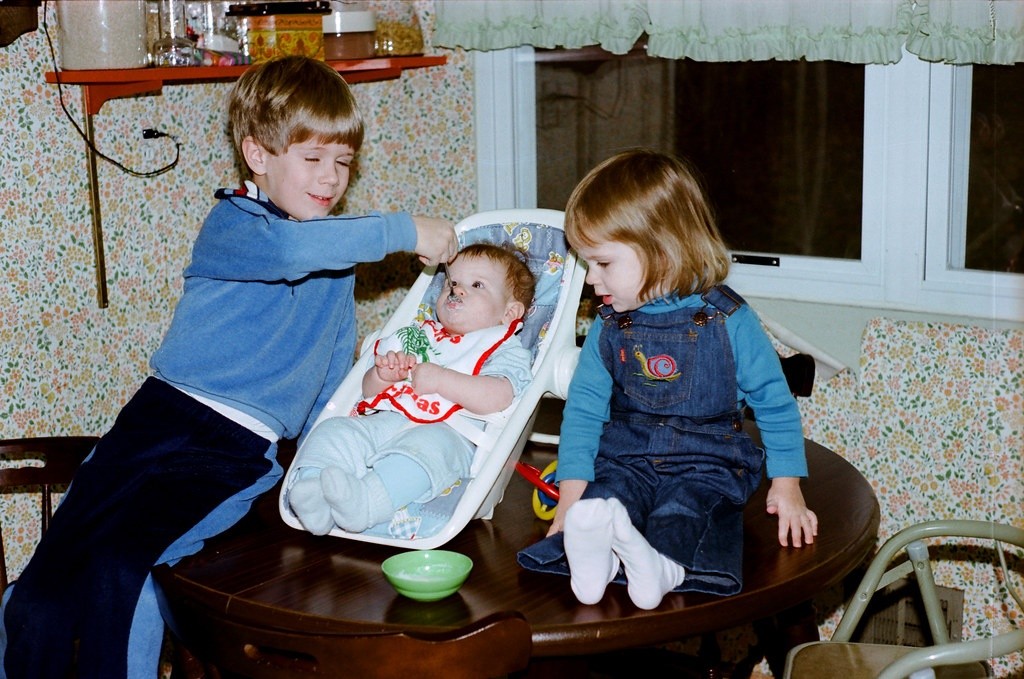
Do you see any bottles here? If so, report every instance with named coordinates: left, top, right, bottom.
left=145, top=0, right=249, bottom=66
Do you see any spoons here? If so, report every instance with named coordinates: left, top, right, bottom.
left=444, top=262, right=463, bottom=303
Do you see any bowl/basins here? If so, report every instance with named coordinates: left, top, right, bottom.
left=382, top=550, right=473, bottom=602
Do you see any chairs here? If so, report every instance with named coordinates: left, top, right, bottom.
left=0, top=436, right=101, bottom=599
left=161, top=605, right=531, bottom=679
left=782, top=520, right=1024, bottom=679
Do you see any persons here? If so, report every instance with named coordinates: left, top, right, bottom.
left=0, top=56, right=458, bottom=678
left=544, top=146, right=818, bottom=610
left=288, top=243, right=539, bottom=537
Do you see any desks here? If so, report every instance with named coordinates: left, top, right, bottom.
left=152, top=399, right=881, bottom=679
